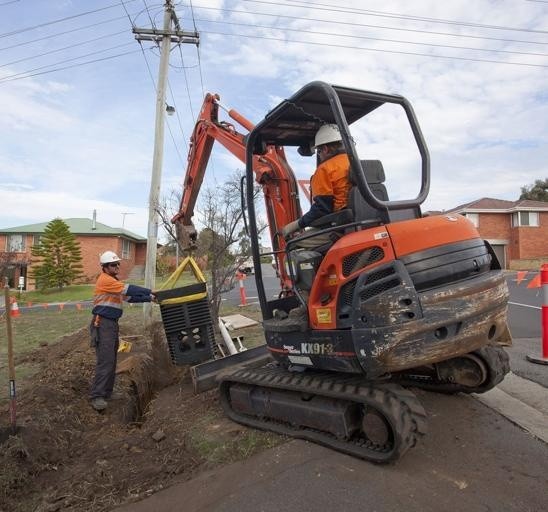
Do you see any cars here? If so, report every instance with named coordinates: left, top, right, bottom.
left=232, top=256, right=254, bottom=273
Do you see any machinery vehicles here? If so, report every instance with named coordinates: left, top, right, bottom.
left=171, top=80, right=517, bottom=464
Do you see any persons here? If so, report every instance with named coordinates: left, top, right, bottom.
left=283, top=124, right=353, bottom=320
left=89, top=251, right=161, bottom=410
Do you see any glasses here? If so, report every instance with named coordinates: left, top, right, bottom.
left=110, top=262, right=120, bottom=267
left=317, top=148, right=323, bottom=155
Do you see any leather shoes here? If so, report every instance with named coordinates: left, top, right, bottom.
left=88, top=396, right=108, bottom=411
left=289, top=305, right=305, bottom=316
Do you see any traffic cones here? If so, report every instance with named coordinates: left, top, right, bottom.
left=7, top=296, right=22, bottom=317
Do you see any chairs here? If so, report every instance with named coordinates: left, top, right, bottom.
left=308, top=160, right=389, bottom=274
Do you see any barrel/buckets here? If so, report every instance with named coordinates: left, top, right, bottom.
left=117, top=339, right=133, bottom=354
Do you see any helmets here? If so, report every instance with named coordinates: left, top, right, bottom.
left=99, top=250, right=122, bottom=266
left=311, top=123, right=342, bottom=149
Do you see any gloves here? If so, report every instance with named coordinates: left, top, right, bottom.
left=282, top=219, right=301, bottom=239
